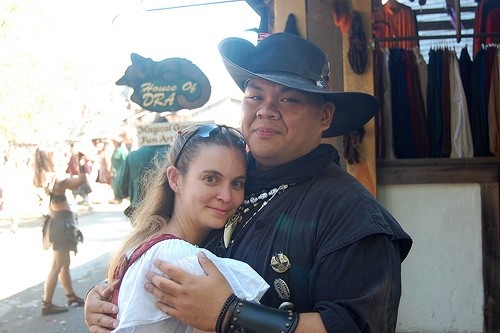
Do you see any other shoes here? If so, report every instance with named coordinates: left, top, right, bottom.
left=87, top=204, right=94, bottom=213
left=108, top=197, right=123, bottom=203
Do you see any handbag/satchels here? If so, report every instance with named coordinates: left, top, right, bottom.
left=42, top=214, right=55, bottom=250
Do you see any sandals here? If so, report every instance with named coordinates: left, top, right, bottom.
left=42, top=293, right=84, bottom=315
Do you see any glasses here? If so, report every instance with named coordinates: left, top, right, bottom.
left=174, top=122, right=246, bottom=168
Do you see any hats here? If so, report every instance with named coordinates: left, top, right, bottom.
left=110, top=135, right=123, bottom=142
left=218, top=32, right=380, bottom=137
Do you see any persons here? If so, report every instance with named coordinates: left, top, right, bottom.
left=0, top=116, right=177, bottom=233
left=84, top=32, right=413, bottom=333
left=33, top=143, right=87, bottom=315
left=106, top=122, right=260, bottom=333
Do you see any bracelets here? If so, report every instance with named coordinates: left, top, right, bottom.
left=215, top=294, right=237, bottom=333
